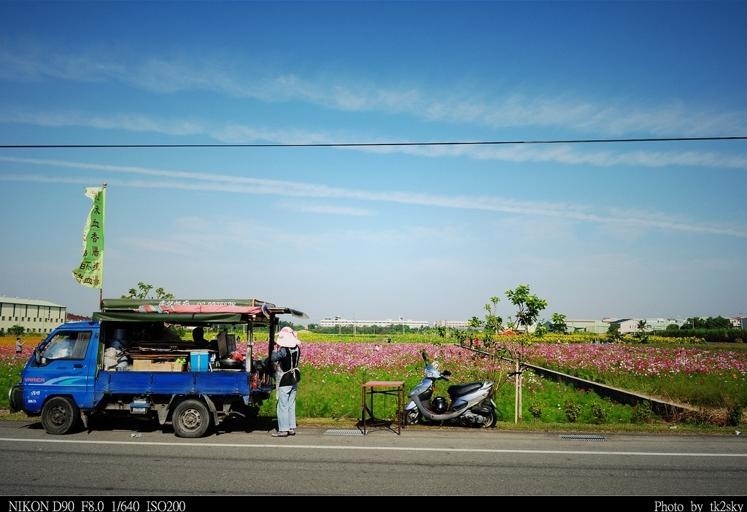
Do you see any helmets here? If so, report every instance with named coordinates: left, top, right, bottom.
left=431, top=397, right=447, bottom=413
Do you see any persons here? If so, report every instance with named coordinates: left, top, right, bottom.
left=15, top=337, right=27, bottom=354
left=192, top=327, right=210, bottom=344
left=263, top=326, right=302, bottom=437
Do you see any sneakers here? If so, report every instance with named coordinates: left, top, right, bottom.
left=272, top=431, right=289, bottom=437
left=290, top=430, right=296, bottom=435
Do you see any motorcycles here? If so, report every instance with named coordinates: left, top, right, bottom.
left=402, top=351, right=497, bottom=430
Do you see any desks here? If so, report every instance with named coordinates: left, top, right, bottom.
left=362, top=381, right=405, bottom=435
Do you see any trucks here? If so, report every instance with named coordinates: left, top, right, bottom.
left=8, top=320, right=277, bottom=439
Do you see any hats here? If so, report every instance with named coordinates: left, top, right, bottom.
left=275, top=326, right=301, bottom=348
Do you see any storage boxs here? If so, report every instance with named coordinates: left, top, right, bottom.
left=190, top=352, right=209, bottom=372
